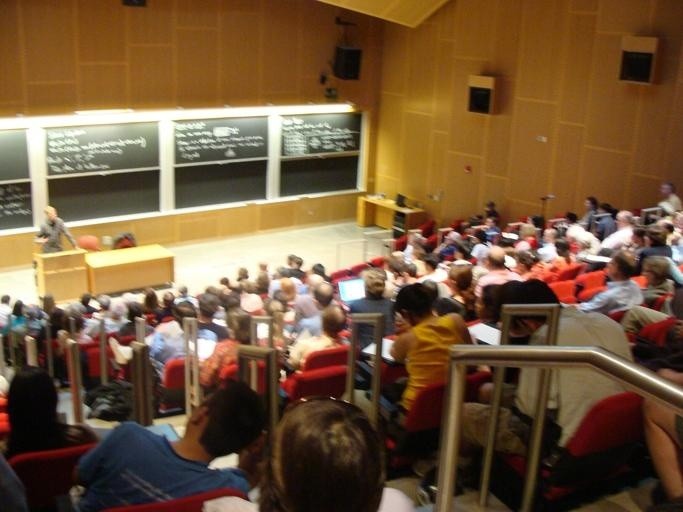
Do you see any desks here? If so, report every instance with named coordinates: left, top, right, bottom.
left=354, top=194, right=425, bottom=238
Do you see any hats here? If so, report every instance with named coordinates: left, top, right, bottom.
left=444, top=231, right=470, bottom=253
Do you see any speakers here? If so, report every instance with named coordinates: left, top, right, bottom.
left=122, top=0, right=147, bottom=7
left=333, top=46, right=363, bottom=80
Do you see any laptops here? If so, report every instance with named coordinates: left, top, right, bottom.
left=338, top=278, right=366, bottom=303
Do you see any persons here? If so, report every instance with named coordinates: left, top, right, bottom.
left=0, top=183, right=683, bottom=512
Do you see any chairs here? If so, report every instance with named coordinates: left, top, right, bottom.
left=0, top=208, right=682, bottom=511
